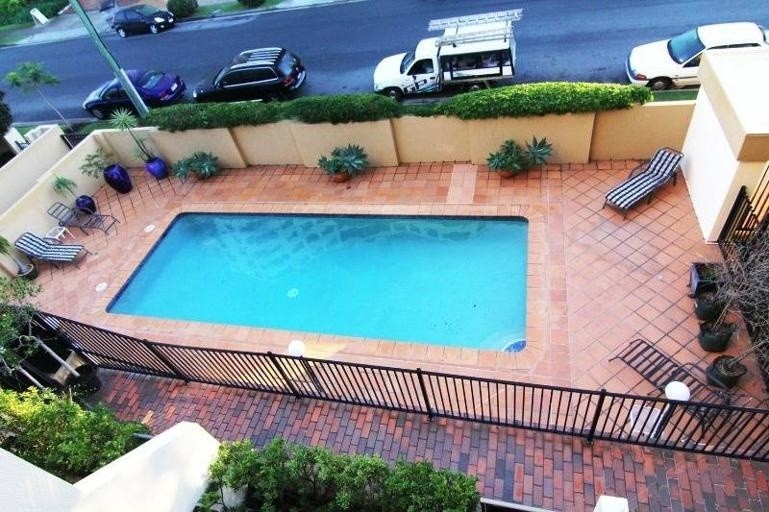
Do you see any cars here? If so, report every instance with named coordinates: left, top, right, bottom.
left=622, top=19, right=769, bottom=89
left=109, top=3, right=176, bottom=39
left=80, top=67, right=187, bottom=122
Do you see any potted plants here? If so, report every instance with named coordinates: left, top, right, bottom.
left=484, top=133, right=553, bottom=178
left=685, top=231, right=769, bottom=390
left=52, top=108, right=222, bottom=213
left=317, top=144, right=373, bottom=182
left=0, top=236, right=102, bottom=395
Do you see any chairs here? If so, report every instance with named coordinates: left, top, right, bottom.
left=607, top=338, right=755, bottom=439
left=13, top=201, right=121, bottom=270
left=601, top=145, right=685, bottom=221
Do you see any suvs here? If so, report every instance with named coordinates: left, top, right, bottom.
left=190, top=44, right=308, bottom=104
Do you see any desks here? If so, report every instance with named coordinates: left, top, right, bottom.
left=614, top=404, right=668, bottom=439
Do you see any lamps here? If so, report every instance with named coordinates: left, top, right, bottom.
left=654, top=379, right=690, bottom=442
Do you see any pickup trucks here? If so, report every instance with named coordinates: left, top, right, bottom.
left=371, top=16, right=520, bottom=101
